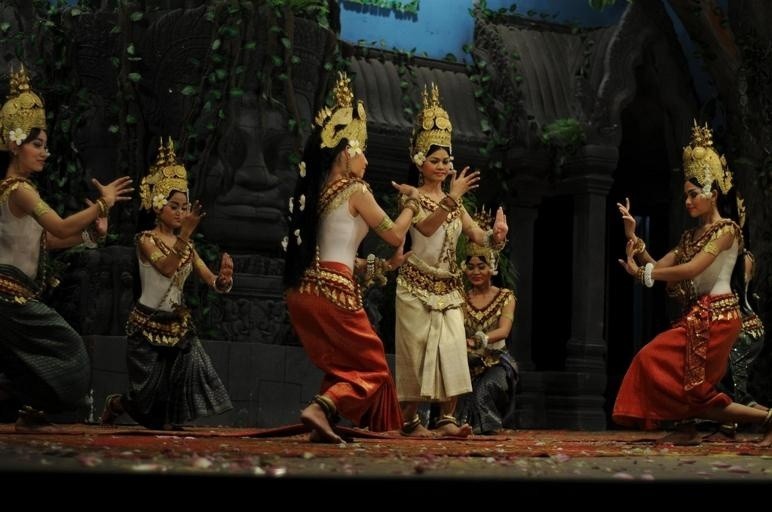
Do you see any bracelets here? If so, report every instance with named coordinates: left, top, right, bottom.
left=404, top=197, right=420, bottom=216
left=483, top=230, right=506, bottom=252
left=95, top=198, right=109, bottom=217
left=473, top=328, right=488, bottom=350
left=171, top=236, right=193, bottom=262
left=81, top=230, right=97, bottom=249
left=212, top=276, right=233, bottom=294
left=634, top=237, right=646, bottom=254
left=366, top=254, right=386, bottom=278
left=439, top=195, right=461, bottom=212
left=637, top=262, right=654, bottom=288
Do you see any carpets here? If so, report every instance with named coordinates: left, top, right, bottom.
left=0, top=423, right=772, bottom=457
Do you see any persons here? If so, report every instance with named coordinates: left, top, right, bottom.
left=282, top=69, right=421, bottom=444
left=96, top=136, right=234, bottom=431
left=395, top=79, right=509, bottom=440
left=613, top=117, right=772, bottom=447
left=702, top=195, right=771, bottom=442
left=429, top=202, right=519, bottom=435
left=0, top=63, right=136, bottom=424
